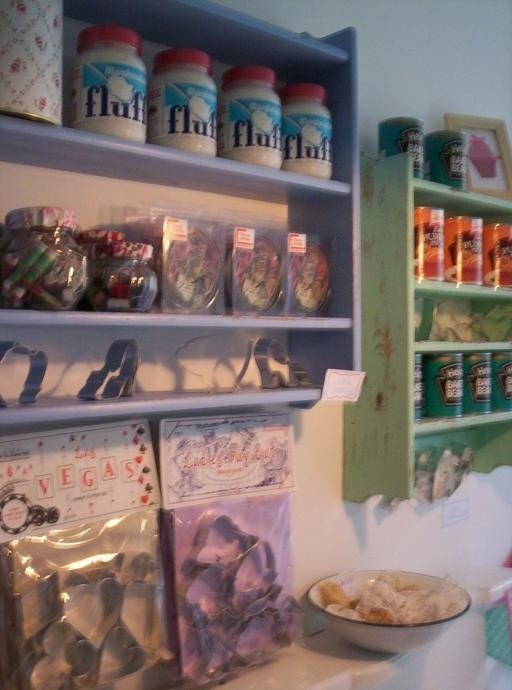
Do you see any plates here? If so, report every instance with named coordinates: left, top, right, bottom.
left=163, top=225, right=333, bottom=315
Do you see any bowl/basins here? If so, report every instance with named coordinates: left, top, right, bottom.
left=304, top=567, right=472, bottom=654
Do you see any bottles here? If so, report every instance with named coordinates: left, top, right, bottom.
left=76, top=25, right=151, bottom=148
left=0, top=204, right=157, bottom=312
left=1, top=0, right=65, bottom=123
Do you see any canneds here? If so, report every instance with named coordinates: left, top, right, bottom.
left=379, top=115, right=425, bottom=179
left=65, top=25, right=148, bottom=144
left=425, top=129, right=469, bottom=190
left=414, top=353, right=512, bottom=422
left=0, top=205, right=159, bottom=313
left=484, top=225, right=512, bottom=286
left=445, top=217, right=483, bottom=284
left=414, top=206, right=445, bottom=283
